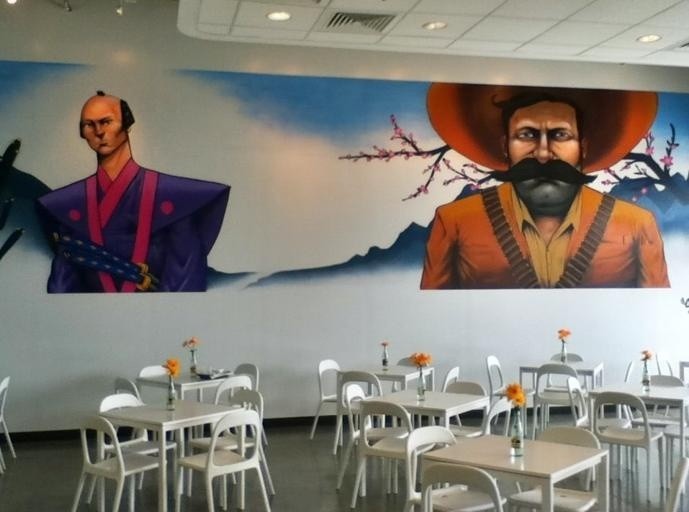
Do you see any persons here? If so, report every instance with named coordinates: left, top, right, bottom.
left=37, top=91, right=230, bottom=292
left=419, top=84, right=673, bottom=289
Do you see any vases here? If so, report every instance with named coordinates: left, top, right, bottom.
left=561, top=342, right=566, bottom=363
left=190, top=351, right=197, bottom=378
left=382, top=346, right=389, bottom=371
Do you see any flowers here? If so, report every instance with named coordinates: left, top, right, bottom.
left=382, top=342, right=387, bottom=346
left=558, top=329, right=571, bottom=342
left=182, top=337, right=197, bottom=351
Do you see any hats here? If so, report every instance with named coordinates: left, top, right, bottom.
left=426, top=81, right=658, bottom=174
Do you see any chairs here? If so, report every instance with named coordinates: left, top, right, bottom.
left=0, top=376, right=17, bottom=470
left=71, top=364, right=276, bottom=511
